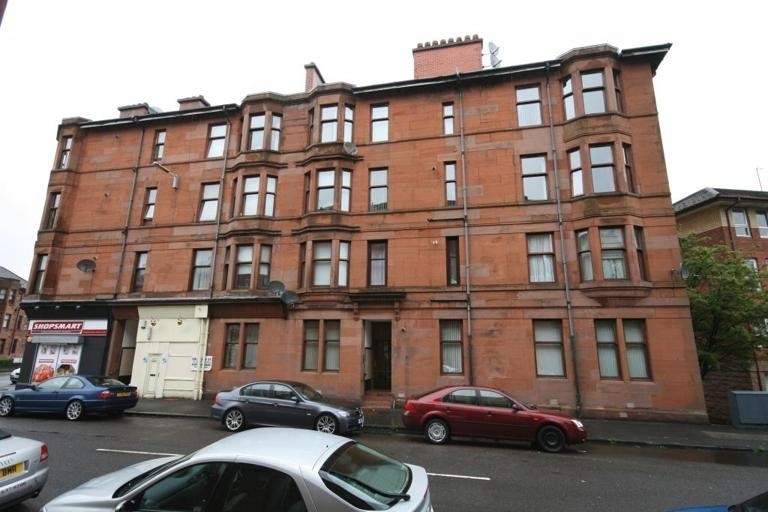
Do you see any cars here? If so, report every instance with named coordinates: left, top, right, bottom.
left=9, top=367, right=20, bottom=383
left=211, top=380, right=365, bottom=434
left=0, top=375, right=139, bottom=421
left=37, top=426, right=435, bottom=512
left=402, top=385, right=587, bottom=453
left=0, top=428, right=51, bottom=512
